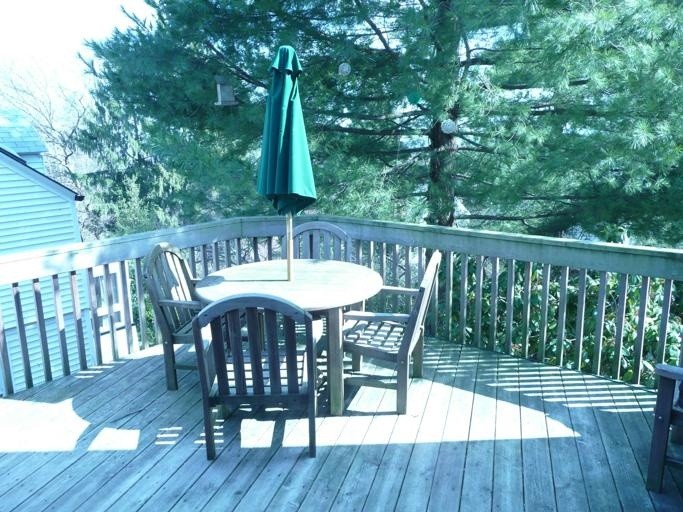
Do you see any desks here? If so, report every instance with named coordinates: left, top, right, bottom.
left=192, top=257, right=386, bottom=418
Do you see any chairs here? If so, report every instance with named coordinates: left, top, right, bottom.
left=190, top=291, right=325, bottom=458
left=329, top=248, right=440, bottom=416
left=278, top=221, right=354, bottom=261
left=146, top=240, right=265, bottom=393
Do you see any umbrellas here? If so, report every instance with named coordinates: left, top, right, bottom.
left=257, top=44, right=318, bottom=282
